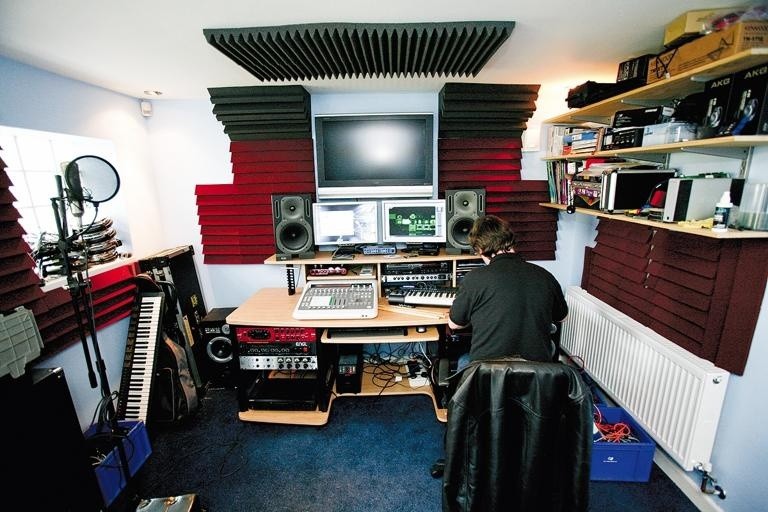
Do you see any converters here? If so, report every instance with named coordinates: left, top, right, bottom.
left=410, top=364, right=420, bottom=371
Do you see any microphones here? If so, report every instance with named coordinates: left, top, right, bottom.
left=59, top=160, right=85, bottom=217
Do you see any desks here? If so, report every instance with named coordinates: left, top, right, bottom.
left=225, top=248, right=516, bottom=426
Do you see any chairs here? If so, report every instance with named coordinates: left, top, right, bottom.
left=430, top=357, right=591, bottom=512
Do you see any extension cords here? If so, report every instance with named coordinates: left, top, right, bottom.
left=406, top=359, right=431, bottom=388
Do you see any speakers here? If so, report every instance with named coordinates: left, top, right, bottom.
left=271, top=192, right=315, bottom=260
left=201, top=306, right=237, bottom=384
left=444, top=188, right=487, bottom=255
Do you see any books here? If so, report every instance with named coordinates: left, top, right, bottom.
left=545, top=120, right=646, bottom=211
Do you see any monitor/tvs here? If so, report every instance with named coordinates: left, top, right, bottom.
left=312, top=201, right=377, bottom=251
left=381, top=199, right=446, bottom=244
left=313, top=113, right=434, bottom=187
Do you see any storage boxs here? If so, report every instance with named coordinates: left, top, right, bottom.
left=647, top=21, right=767, bottom=82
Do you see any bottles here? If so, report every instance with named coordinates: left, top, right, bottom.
left=710, top=191, right=733, bottom=233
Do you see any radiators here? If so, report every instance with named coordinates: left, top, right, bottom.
left=558, top=284, right=731, bottom=474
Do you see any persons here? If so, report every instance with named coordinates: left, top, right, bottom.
left=449, top=214, right=569, bottom=375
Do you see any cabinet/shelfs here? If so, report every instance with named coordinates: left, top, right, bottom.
left=538, top=47, right=768, bottom=239
